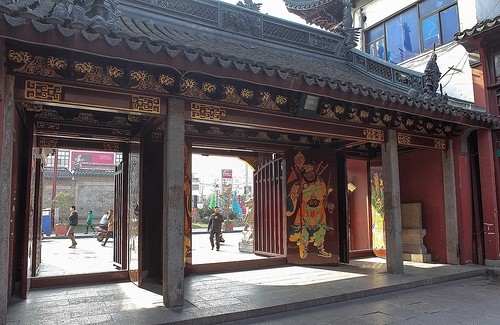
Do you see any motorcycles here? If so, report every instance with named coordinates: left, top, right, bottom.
left=93, top=223, right=113, bottom=242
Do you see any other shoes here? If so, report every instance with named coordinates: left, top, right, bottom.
left=211, top=247, right=214, bottom=250
left=101, top=244, right=106, bottom=246
left=72, top=242, right=77, bottom=246
left=216, top=248, right=219, bottom=251
left=69, top=246, right=75, bottom=248
left=84, top=232, right=88, bottom=234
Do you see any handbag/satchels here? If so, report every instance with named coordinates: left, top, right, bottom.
left=87, top=221, right=91, bottom=225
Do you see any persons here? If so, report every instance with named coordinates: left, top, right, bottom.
left=65, top=205, right=79, bottom=249
left=84, top=210, right=96, bottom=234
left=100, top=208, right=114, bottom=246
left=370, top=174, right=387, bottom=250
left=207, top=206, right=224, bottom=251
